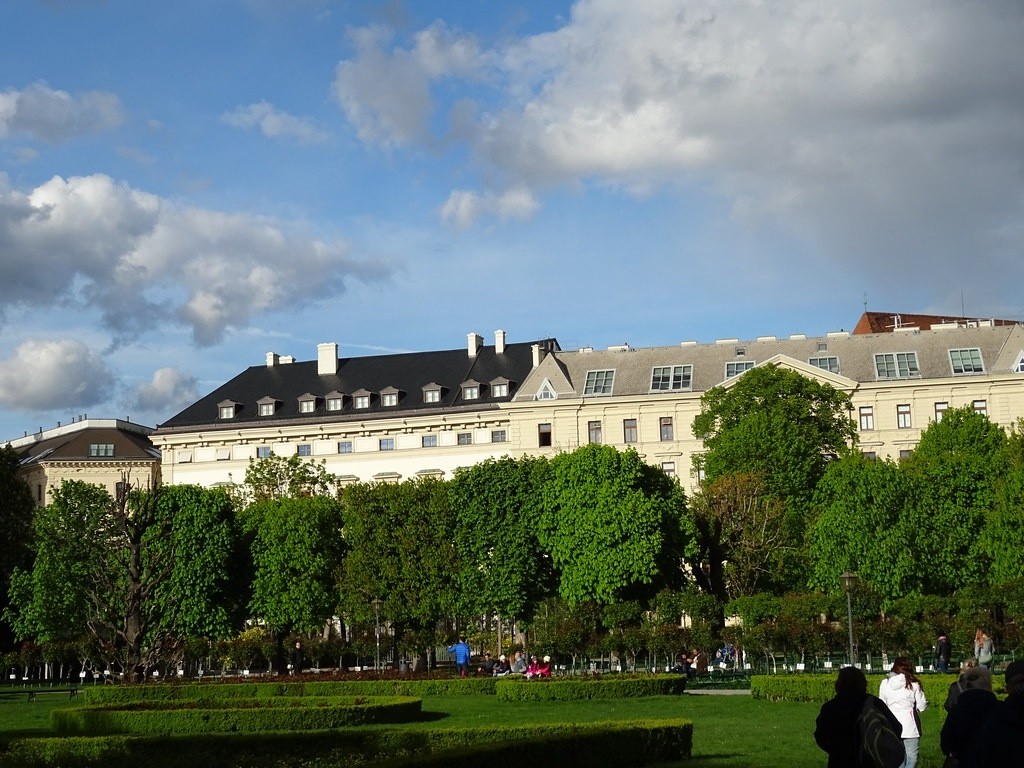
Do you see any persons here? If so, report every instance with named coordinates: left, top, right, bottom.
left=447, top=636, right=710, bottom=685
left=815, top=626, right=1024, bottom=768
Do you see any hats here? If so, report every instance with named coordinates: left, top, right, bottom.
left=499, top=654, right=506, bottom=663
left=543, top=656, right=551, bottom=662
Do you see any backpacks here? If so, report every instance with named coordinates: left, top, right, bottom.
left=853, top=694, right=907, bottom=768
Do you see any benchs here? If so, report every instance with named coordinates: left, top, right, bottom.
left=692, top=662, right=761, bottom=688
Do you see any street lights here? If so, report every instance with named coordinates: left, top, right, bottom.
left=371, top=595, right=385, bottom=680
left=838, top=569, right=859, bottom=668
